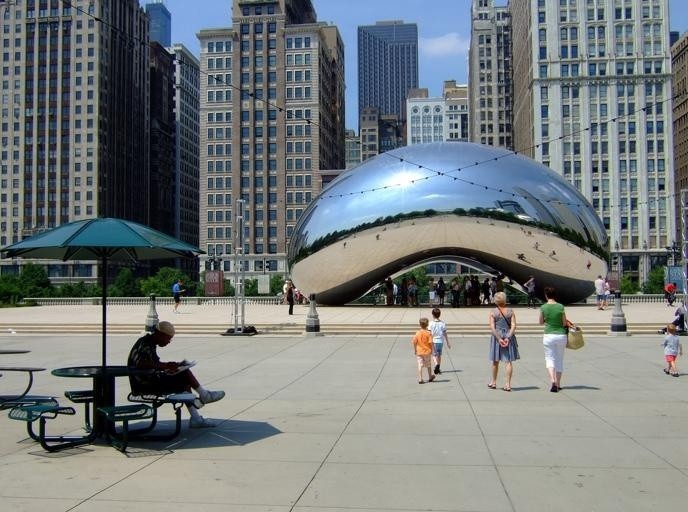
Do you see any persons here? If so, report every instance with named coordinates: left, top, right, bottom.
left=660, top=324, right=683, bottom=378
left=594, top=275, right=606, bottom=310
left=524, top=277, right=536, bottom=308
left=665, top=282, right=677, bottom=307
left=128, top=322, right=226, bottom=428
left=412, top=319, right=436, bottom=383
left=173, top=278, right=186, bottom=314
left=658, top=302, right=685, bottom=335
left=487, top=292, right=520, bottom=392
left=429, top=308, right=450, bottom=374
left=539, top=285, right=584, bottom=393
left=380, top=274, right=503, bottom=309
left=282, top=279, right=303, bottom=315
left=602, top=279, right=611, bottom=310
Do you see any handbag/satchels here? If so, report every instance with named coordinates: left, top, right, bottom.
left=566, top=324, right=584, bottom=350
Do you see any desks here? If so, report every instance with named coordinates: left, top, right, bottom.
left=0, top=349, right=31, bottom=358
left=51, top=365, right=130, bottom=446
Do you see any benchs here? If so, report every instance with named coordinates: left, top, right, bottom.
left=0, top=367, right=47, bottom=408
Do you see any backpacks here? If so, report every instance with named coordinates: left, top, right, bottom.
left=287, top=289, right=292, bottom=303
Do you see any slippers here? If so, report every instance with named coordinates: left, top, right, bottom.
left=550, top=382, right=562, bottom=392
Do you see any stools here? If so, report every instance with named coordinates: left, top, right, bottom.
left=8, top=390, right=196, bottom=453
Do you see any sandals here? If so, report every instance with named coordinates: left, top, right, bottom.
left=487, top=384, right=496, bottom=389
left=503, top=386, right=512, bottom=392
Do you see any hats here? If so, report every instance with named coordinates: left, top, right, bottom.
left=154, top=321, right=176, bottom=339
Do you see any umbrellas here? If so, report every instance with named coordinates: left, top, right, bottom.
left=0, top=218, right=207, bottom=373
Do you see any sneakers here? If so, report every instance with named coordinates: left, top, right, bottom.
left=200, top=391, right=225, bottom=404
left=418, top=375, right=435, bottom=384
left=663, top=368, right=679, bottom=377
left=189, top=416, right=215, bottom=427
left=435, top=364, right=440, bottom=375
